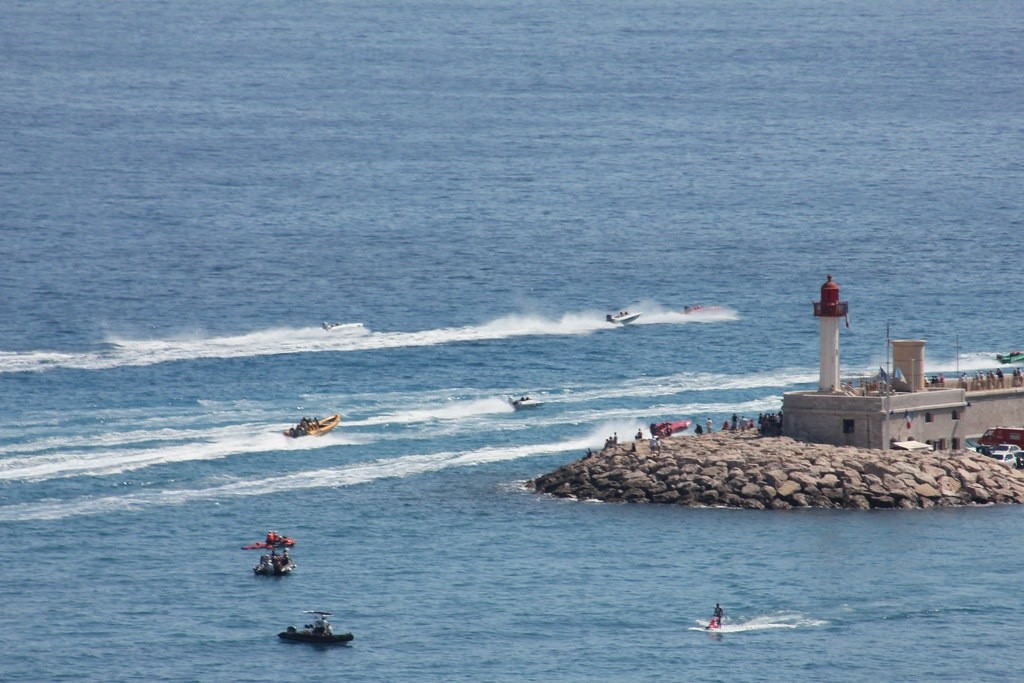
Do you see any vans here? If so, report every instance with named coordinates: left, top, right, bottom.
left=978, top=427, right=1024, bottom=450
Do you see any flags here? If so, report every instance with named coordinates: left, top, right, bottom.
left=880, top=367, right=891, bottom=384
left=894, top=366, right=906, bottom=382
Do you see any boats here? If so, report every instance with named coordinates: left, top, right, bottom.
left=278, top=611, right=353, bottom=645
left=652, top=419, right=692, bottom=435
left=512, top=397, right=545, bottom=409
left=684, top=304, right=719, bottom=315
left=254, top=552, right=293, bottom=576
left=997, top=352, right=1024, bottom=364
left=242, top=531, right=295, bottom=550
left=606, top=311, right=643, bottom=325
left=283, top=414, right=339, bottom=437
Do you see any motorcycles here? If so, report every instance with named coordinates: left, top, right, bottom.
left=706, top=614, right=721, bottom=631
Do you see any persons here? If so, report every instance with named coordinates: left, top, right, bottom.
left=298, top=416, right=320, bottom=431
left=830, top=379, right=896, bottom=396
left=519, top=396, right=529, bottom=401
left=321, top=321, right=340, bottom=328
left=713, top=603, right=723, bottom=628
left=585, top=411, right=783, bottom=458
left=259, top=530, right=290, bottom=570
left=924, top=350, right=1024, bottom=391
left=303, top=616, right=333, bottom=636
left=618, top=309, right=629, bottom=315
left=692, top=305, right=700, bottom=309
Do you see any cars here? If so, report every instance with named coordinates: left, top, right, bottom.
left=990, top=453, right=1017, bottom=469
left=1014, top=451, right=1024, bottom=468
left=994, top=444, right=1021, bottom=457
left=976, top=444, right=995, bottom=457
